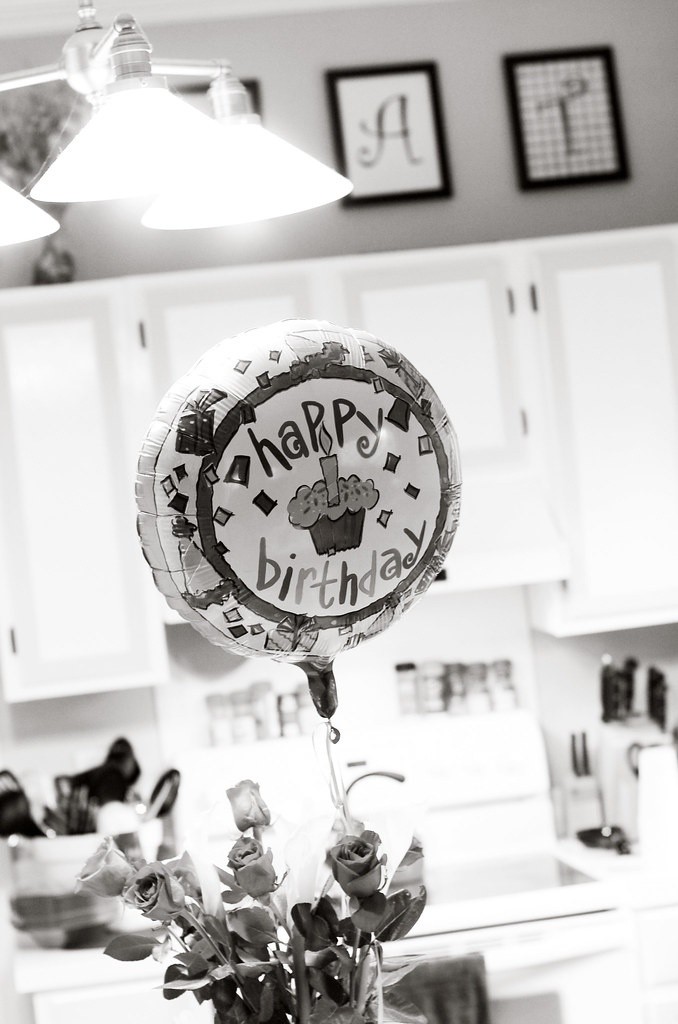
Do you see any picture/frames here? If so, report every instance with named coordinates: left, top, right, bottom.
left=505, top=49, right=632, bottom=191
left=320, top=65, right=452, bottom=207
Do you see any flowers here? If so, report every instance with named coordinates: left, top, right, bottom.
left=80, top=780, right=426, bottom=1024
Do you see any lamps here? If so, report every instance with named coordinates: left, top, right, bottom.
left=0, top=0, right=355, bottom=243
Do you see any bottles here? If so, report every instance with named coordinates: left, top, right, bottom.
left=397, top=658, right=516, bottom=716
left=204, top=681, right=310, bottom=744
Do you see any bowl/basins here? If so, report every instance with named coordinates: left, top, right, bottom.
left=0, top=820, right=166, bottom=939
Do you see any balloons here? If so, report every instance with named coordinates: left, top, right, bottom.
left=134, top=317, right=462, bottom=746
